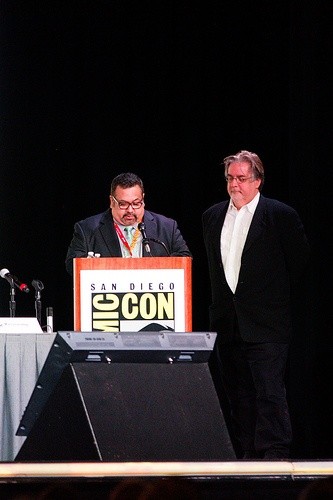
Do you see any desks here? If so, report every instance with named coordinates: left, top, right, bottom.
left=0, top=333, right=57, bottom=461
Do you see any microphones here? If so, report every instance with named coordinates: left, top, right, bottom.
left=0, top=268, right=30, bottom=294
left=138, top=223, right=151, bottom=253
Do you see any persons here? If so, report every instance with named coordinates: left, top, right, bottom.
left=190, top=150, right=311, bottom=463
left=65, top=171, right=193, bottom=278
